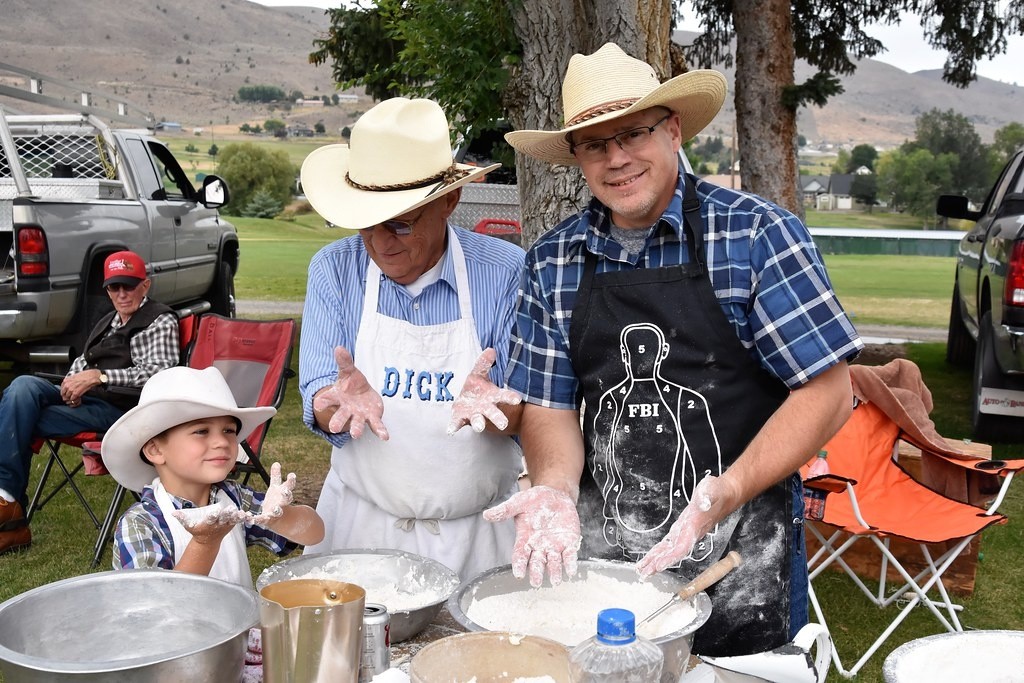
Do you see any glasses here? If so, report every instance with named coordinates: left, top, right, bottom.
left=106, top=283, right=135, bottom=292
left=360, top=203, right=428, bottom=236
left=569, top=116, right=670, bottom=161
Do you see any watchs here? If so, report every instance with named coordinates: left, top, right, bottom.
left=100, top=369, right=109, bottom=391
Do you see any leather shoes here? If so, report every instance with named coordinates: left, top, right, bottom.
left=0, top=496, right=25, bottom=529
left=0, top=526, right=30, bottom=555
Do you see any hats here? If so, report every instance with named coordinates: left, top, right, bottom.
left=100, top=366, right=277, bottom=494
left=300, top=98, right=504, bottom=229
left=103, top=251, right=147, bottom=287
left=504, top=42, right=728, bottom=166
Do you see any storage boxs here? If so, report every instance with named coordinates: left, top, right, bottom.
left=803, top=442, right=981, bottom=592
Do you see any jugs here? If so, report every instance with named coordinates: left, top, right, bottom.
left=258, top=579, right=365, bottom=683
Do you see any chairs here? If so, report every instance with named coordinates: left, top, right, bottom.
left=92, top=311, right=301, bottom=570
left=27, top=299, right=213, bottom=553
left=799, top=358, right=1024, bottom=676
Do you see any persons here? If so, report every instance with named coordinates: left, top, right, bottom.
left=99, top=366, right=326, bottom=627
left=0, top=252, right=183, bottom=553
left=298, top=95, right=527, bottom=601
left=478, top=41, right=867, bottom=657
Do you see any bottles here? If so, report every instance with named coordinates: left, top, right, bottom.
left=803, top=451, right=830, bottom=521
left=567, top=608, right=664, bottom=683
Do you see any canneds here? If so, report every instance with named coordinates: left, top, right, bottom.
left=357, top=603, right=391, bottom=683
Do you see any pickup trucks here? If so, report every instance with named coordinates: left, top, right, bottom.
left=446, top=118, right=696, bottom=247
left=0, top=112, right=240, bottom=364
left=936, top=145, right=1024, bottom=445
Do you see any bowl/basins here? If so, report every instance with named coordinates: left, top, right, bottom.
left=255, top=549, right=459, bottom=643
left=0, top=568, right=258, bottom=683
left=410, top=632, right=572, bottom=683
left=447, top=558, right=712, bottom=683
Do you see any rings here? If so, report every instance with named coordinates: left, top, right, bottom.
left=72, top=400, right=74, bottom=404
